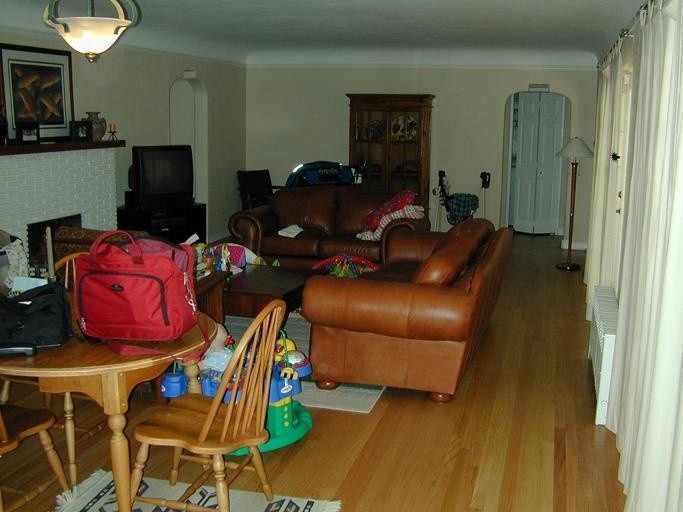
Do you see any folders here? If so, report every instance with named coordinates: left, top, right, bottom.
left=589, top=286, right=619, bottom=425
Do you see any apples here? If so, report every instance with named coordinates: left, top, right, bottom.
left=110, top=123, right=115, bottom=132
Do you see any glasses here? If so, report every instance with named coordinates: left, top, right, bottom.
left=40, top=0, right=143, bottom=61
left=555, top=138, right=594, bottom=271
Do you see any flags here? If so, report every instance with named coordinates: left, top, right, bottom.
left=81, top=112, right=107, bottom=142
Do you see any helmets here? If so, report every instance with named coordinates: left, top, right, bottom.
left=365, top=120, right=384, bottom=141
left=391, top=114, right=418, bottom=141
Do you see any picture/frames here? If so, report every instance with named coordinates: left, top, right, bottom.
left=0, top=292, right=218, bottom=512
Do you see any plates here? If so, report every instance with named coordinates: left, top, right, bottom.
left=117, top=203, right=205, bottom=246
left=345, top=94, right=435, bottom=219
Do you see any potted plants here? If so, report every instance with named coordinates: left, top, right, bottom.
left=0, top=44, right=75, bottom=140
left=15, top=121, right=40, bottom=145
left=70, top=121, right=93, bottom=142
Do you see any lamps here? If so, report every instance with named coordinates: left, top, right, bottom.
left=44, top=253, right=87, bottom=291
left=129, top=299, right=289, bottom=512
left=0, top=404, right=72, bottom=512
left=237, top=169, right=285, bottom=211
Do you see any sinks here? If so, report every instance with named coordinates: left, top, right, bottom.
left=301, top=218, right=515, bottom=403
left=227, top=184, right=431, bottom=266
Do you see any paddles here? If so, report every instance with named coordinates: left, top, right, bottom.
left=221, top=314, right=385, bottom=414
left=54, top=468, right=344, bottom=512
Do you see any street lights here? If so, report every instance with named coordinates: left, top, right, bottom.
left=0, top=281, right=70, bottom=348
left=287, top=161, right=355, bottom=188
left=73, top=230, right=197, bottom=341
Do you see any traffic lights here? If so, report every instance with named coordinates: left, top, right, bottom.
left=273, top=187, right=337, bottom=236
left=336, top=186, right=392, bottom=234
left=414, top=221, right=488, bottom=285
